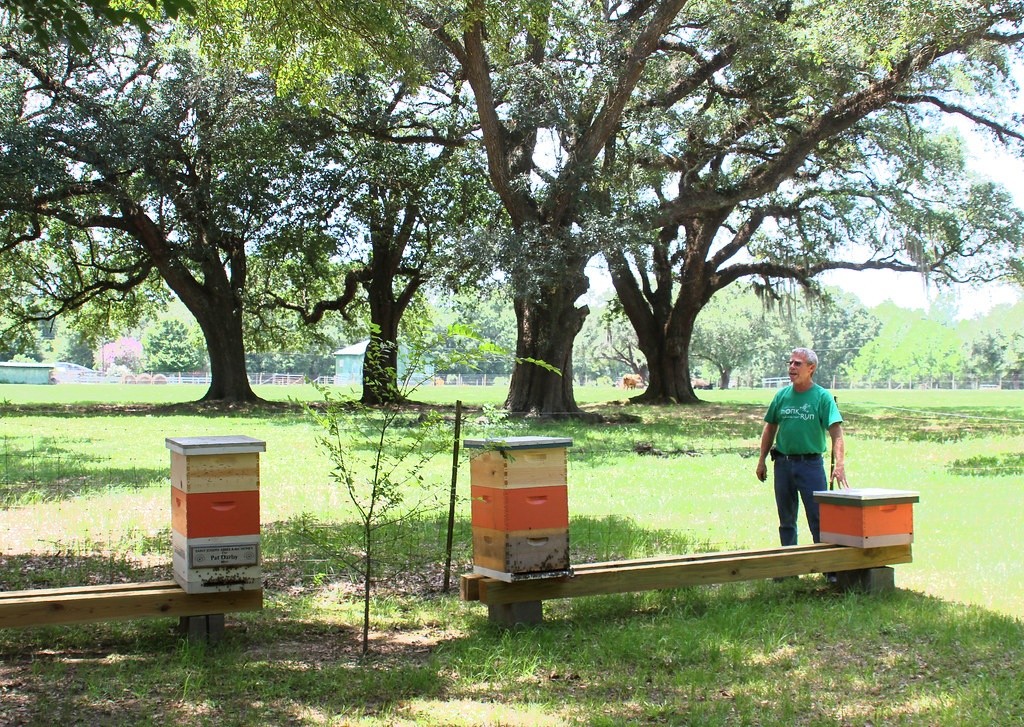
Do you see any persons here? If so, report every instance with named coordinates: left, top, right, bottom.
left=756, top=347, right=848, bottom=586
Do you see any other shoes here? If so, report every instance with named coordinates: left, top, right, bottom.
left=827, top=577, right=836, bottom=583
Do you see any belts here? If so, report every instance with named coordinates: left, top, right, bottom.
left=780, top=454, right=822, bottom=460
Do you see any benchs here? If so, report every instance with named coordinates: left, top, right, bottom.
left=458, top=542, right=914, bottom=626
left=0, top=578, right=266, bottom=646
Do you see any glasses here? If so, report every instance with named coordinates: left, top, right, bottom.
left=788, top=360, right=808, bottom=367
